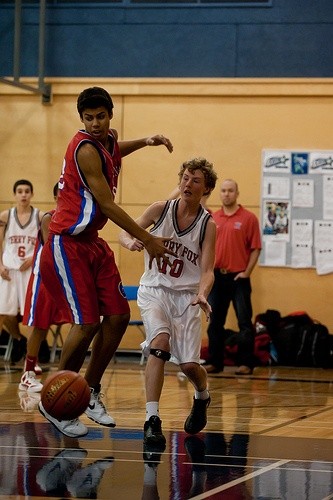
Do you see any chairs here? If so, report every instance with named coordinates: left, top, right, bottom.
left=111, top=286, right=148, bottom=366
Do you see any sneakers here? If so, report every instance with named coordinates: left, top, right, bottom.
left=37, top=400, right=89, bottom=438
left=184, top=436, right=209, bottom=473
left=84, top=384, right=116, bottom=428
left=142, top=444, right=167, bottom=470
left=65, top=456, right=115, bottom=498
left=18, top=370, right=44, bottom=393
left=33, top=363, right=42, bottom=375
left=184, top=395, right=212, bottom=435
left=143, top=415, right=166, bottom=444
left=35, top=446, right=88, bottom=493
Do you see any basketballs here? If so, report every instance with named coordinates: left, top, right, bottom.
left=40, top=370, right=91, bottom=421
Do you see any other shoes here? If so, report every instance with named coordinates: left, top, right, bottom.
left=204, top=364, right=224, bottom=374
left=38, top=339, right=51, bottom=364
left=234, top=365, right=253, bottom=375
left=10, top=334, right=28, bottom=362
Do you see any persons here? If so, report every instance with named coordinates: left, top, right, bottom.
left=39, top=86, right=179, bottom=438
left=19, top=182, right=58, bottom=394
left=11, top=432, right=256, bottom=500
left=119, top=160, right=217, bottom=447
left=0, top=179, right=45, bottom=373
left=205, top=179, right=262, bottom=375
